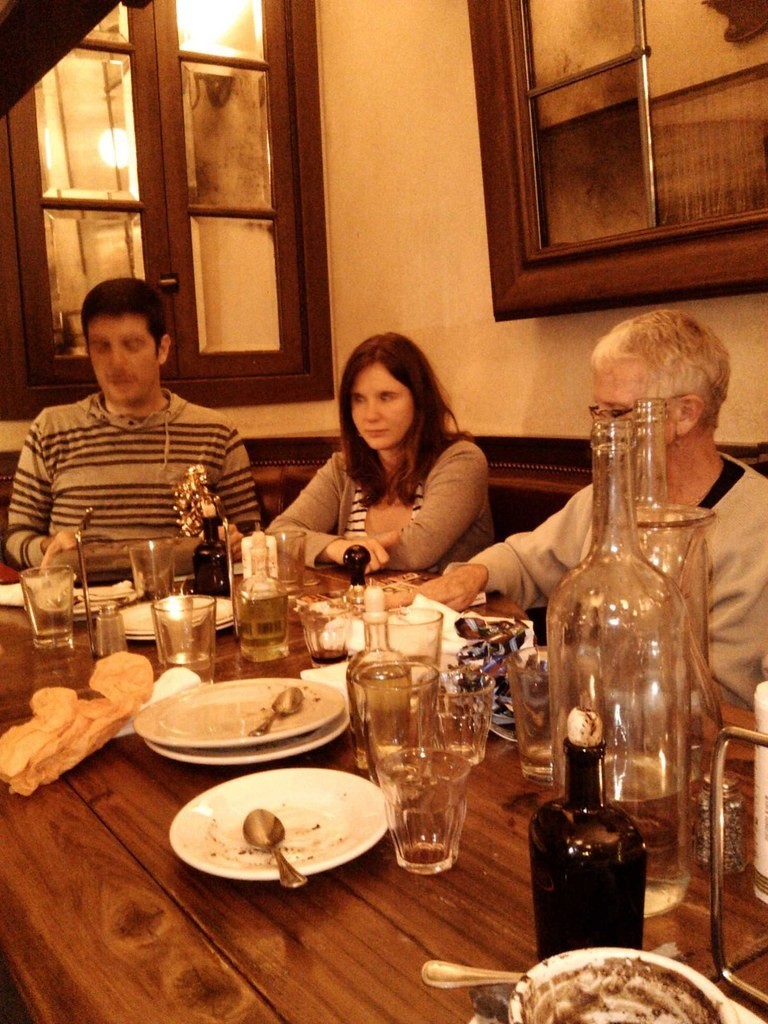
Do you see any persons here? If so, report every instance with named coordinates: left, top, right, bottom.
left=0, top=278, right=265, bottom=571
left=384, top=308, right=768, bottom=711
left=266, top=332, right=496, bottom=575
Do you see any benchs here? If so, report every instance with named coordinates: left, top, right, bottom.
left=0, top=461, right=595, bottom=557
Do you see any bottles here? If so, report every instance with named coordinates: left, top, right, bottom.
left=630, top=398, right=670, bottom=512
left=347, top=586, right=412, bottom=772
left=546, top=420, right=693, bottom=920
left=91, top=601, right=128, bottom=660
left=192, top=518, right=231, bottom=599
left=693, top=771, right=747, bottom=875
left=527, top=706, right=647, bottom=963
left=235, top=545, right=290, bottom=663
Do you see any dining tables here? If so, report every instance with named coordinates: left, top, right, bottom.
left=0, top=563, right=768, bottom=1024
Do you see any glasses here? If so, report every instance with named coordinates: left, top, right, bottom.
left=588, top=393, right=691, bottom=421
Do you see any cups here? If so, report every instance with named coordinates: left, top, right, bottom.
left=635, top=503, right=715, bottom=754
left=375, top=749, right=472, bottom=876
left=418, top=670, right=497, bottom=766
left=269, top=530, right=307, bottom=599
left=241, top=536, right=278, bottom=581
left=363, top=609, right=443, bottom=717
left=151, top=596, right=215, bottom=687
left=128, top=538, right=175, bottom=601
left=506, top=648, right=599, bottom=785
left=754, top=680, right=768, bottom=904
left=298, top=601, right=354, bottom=669
left=352, top=660, right=440, bottom=798
left=19, top=565, right=73, bottom=649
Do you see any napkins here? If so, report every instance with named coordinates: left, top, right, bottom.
left=0, top=595, right=534, bottom=796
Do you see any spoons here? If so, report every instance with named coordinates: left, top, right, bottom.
left=242, top=809, right=308, bottom=889
left=248, top=686, right=304, bottom=739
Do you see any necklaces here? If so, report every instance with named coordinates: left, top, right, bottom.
left=690, top=479, right=715, bottom=506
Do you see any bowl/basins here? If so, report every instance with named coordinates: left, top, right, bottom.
left=507, top=947, right=740, bottom=1024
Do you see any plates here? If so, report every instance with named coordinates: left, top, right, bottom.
left=168, top=767, right=389, bottom=881
left=131, top=678, right=350, bottom=766
left=101, top=596, right=235, bottom=640
left=465, top=999, right=766, bottom=1024
left=35, top=584, right=137, bottom=622
